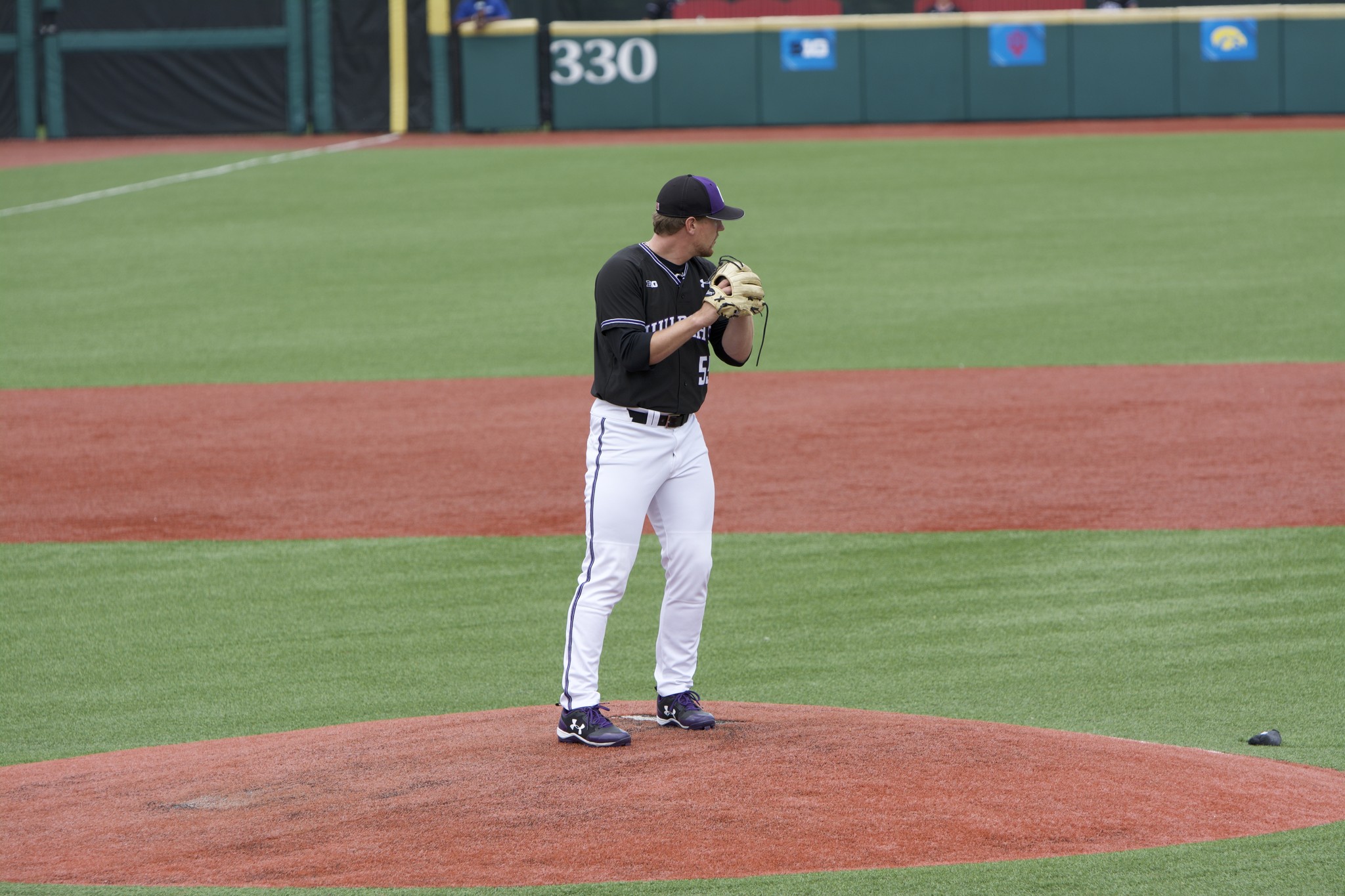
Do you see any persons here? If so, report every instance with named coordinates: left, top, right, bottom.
left=554, top=174, right=769, bottom=747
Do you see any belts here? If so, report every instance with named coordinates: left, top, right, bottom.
left=627, top=408, right=689, bottom=429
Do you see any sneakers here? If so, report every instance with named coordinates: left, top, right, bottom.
left=557, top=704, right=632, bottom=748
left=655, top=687, right=717, bottom=732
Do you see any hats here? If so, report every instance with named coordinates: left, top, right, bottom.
left=655, top=174, right=744, bottom=221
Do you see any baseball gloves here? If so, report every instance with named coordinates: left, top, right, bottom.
left=702, top=261, right=766, bottom=320
